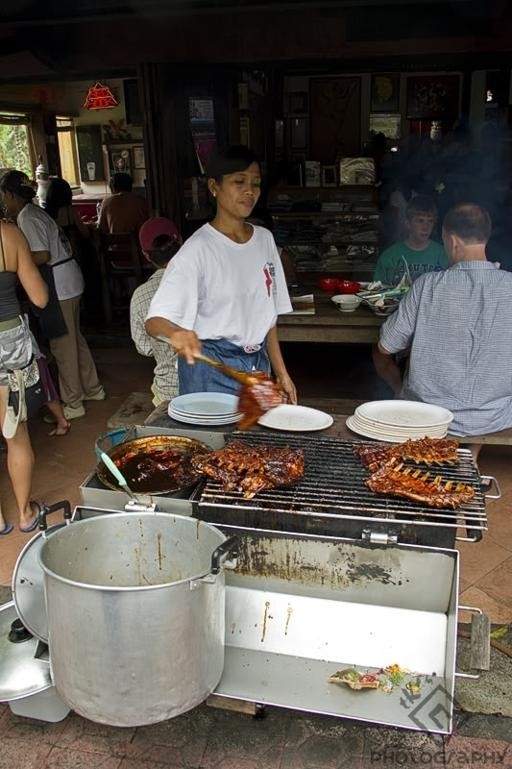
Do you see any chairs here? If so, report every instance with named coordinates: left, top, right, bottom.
left=98, top=228, right=142, bottom=324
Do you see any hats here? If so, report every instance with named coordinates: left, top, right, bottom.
left=139, top=216, right=179, bottom=253
left=2, top=368, right=26, bottom=439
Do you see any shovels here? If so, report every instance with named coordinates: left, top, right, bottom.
left=157, top=336, right=288, bottom=411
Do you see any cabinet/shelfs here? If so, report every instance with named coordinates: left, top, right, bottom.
left=270, top=184, right=382, bottom=272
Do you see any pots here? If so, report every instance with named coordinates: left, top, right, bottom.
left=93, top=431, right=213, bottom=496
left=37, top=499, right=240, bottom=731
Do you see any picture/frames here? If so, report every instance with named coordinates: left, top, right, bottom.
left=370, top=72, right=399, bottom=114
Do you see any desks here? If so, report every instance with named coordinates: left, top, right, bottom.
left=273, top=296, right=386, bottom=345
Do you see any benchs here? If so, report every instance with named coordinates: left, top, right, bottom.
left=104, top=391, right=512, bottom=468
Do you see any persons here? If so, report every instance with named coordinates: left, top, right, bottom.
left=388, top=174, right=419, bottom=239
left=437, top=123, right=471, bottom=165
left=372, top=197, right=449, bottom=400
left=128, top=215, right=185, bottom=423
left=0, top=171, right=147, bottom=435
left=141, top=143, right=298, bottom=407
left=373, top=200, right=512, bottom=463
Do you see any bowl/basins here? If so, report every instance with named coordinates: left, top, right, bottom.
left=337, top=279, right=361, bottom=294
left=320, top=278, right=340, bottom=290
left=369, top=298, right=401, bottom=317
left=331, top=295, right=362, bottom=312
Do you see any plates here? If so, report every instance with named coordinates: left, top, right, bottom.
left=292, top=260, right=376, bottom=273
left=346, top=398, right=454, bottom=443
left=165, top=391, right=249, bottom=425
left=283, top=294, right=316, bottom=316
left=257, top=404, right=334, bottom=434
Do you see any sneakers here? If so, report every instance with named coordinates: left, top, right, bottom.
left=84, top=385, right=107, bottom=402
left=43, top=403, right=85, bottom=424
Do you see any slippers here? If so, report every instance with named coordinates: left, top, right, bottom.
left=21, top=496, right=46, bottom=532
